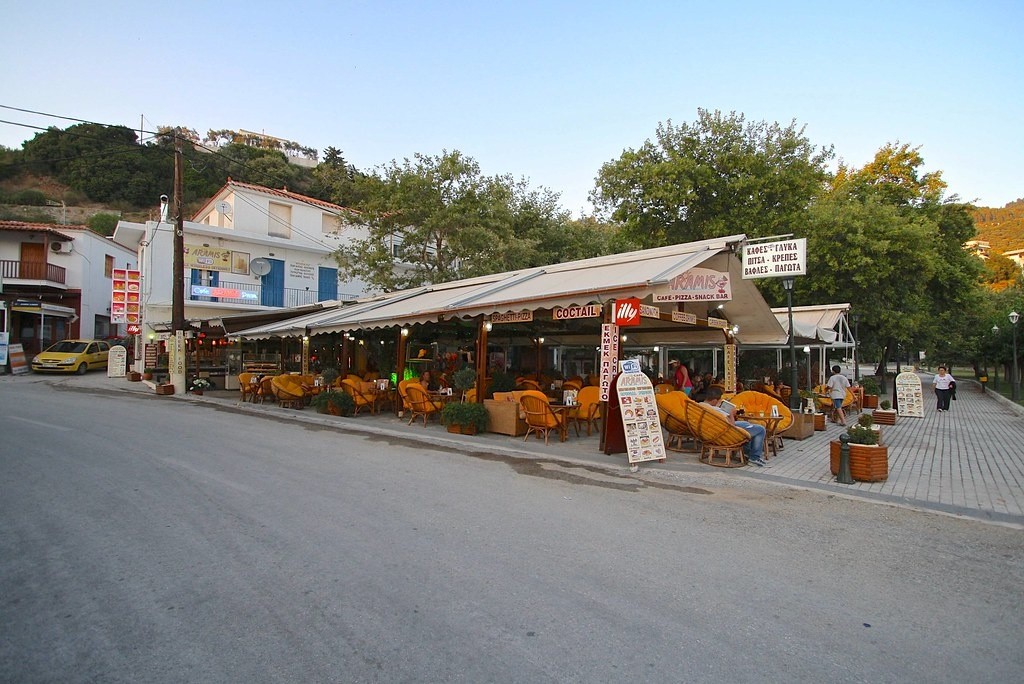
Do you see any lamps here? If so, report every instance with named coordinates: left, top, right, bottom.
left=302, top=335, right=309, bottom=341
left=733, top=324, right=739, bottom=335
left=234, top=339, right=238, bottom=343
left=485, top=320, right=492, bottom=332
left=622, top=335, right=628, bottom=343
left=29, top=235, right=33, bottom=239
left=401, top=326, right=409, bottom=338
left=653, top=345, right=660, bottom=352
left=539, top=337, right=545, bottom=344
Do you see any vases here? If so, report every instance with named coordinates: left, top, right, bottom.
left=192, top=391, right=203, bottom=395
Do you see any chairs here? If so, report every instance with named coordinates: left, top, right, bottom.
left=563, top=382, right=580, bottom=391
left=271, top=376, right=304, bottom=408
left=683, top=399, right=751, bottom=469
left=516, top=376, right=525, bottom=382
left=398, top=380, right=412, bottom=421
left=564, top=386, right=601, bottom=436
left=521, top=380, right=542, bottom=392
left=340, top=379, right=377, bottom=418
left=347, top=375, right=362, bottom=386
left=406, top=383, right=442, bottom=429
left=302, top=375, right=320, bottom=406
left=519, top=390, right=567, bottom=447
left=567, top=378, right=583, bottom=387
left=710, top=384, right=726, bottom=393
left=655, top=391, right=701, bottom=453
left=589, top=376, right=600, bottom=387
left=762, top=385, right=790, bottom=408
left=410, top=378, right=420, bottom=384
left=655, top=384, right=674, bottom=395
left=256, top=376, right=275, bottom=405
left=238, top=372, right=258, bottom=402
left=729, top=390, right=795, bottom=452
left=465, top=388, right=477, bottom=403
left=814, top=384, right=858, bottom=423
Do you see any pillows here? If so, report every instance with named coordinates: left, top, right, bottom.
left=360, top=381, right=375, bottom=395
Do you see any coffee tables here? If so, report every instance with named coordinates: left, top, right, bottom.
left=550, top=401, right=582, bottom=439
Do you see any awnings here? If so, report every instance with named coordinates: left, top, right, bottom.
left=11, top=301, right=75, bottom=351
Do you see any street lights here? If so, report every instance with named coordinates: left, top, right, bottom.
left=851, top=309, right=861, bottom=381
left=782, top=276, right=801, bottom=409
left=992, top=324, right=1000, bottom=392
left=1008, top=309, right=1020, bottom=401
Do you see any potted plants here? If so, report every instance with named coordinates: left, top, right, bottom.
left=440, top=401, right=491, bottom=437
left=850, top=413, right=882, bottom=445
left=142, top=368, right=153, bottom=380
left=310, top=389, right=355, bottom=417
left=156, top=382, right=174, bottom=395
left=859, top=377, right=882, bottom=409
left=126, top=371, right=142, bottom=381
left=829, top=425, right=888, bottom=483
left=872, top=399, right=897, bottom=426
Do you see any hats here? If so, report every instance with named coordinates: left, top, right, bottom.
left=668, top=359, right=679, bottom=364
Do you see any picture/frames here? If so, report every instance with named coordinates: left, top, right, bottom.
left=405, top=342, right=438, bottom=363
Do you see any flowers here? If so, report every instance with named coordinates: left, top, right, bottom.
left=190, top=378, right=211, bottom=392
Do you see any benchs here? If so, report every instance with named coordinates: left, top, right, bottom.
left=900, top=365, right=915, bottom=373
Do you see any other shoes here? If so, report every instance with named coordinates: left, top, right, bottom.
left=744, top=451, right=767, bottom=466
left=938, top=408, right=947, bottom=412
left=837, top=422, right=847, bottom=426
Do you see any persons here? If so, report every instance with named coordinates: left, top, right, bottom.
left=669, top=357, right=692, bottom=396
left=689, top=372, right=744, bottom=394
left=699, top=387, right=771, bottom=468
left=931, top=366, right=956, bottom=412
left=826, top=366, right=858, bottom=426
left=420, top=371, right=440, bottom=391
left=773, top=380, right=789, bottom=407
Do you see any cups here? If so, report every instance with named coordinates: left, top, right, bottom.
left=736, top=404, right=745, bottom=415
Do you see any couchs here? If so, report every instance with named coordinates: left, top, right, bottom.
left=481, top=391, right=562, bottom=437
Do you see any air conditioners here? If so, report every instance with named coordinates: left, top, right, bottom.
left=49, top=241, right=71, bottom=253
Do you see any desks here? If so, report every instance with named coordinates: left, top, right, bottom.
left=736, top=412, right=784, bottom=460
left=783, top=409, right=815, bottom=441
left=804, top=413, right=828, bottom=431
left=369, top=387, right=398, bottom=416
left=248, top=383, right=260, bottom=402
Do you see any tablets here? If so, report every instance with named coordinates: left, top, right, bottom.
left=718, top=399, right=736, bottom=416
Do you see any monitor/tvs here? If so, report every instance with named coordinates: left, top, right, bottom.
left=406, top=343, right=438, bottom=362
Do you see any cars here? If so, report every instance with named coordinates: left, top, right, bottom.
left=32, top=339, right=111, bottom=375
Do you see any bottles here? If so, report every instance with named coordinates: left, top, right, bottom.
left=759, top=405, right=764, bottom=418
left=819, top=386, right=822, bottom=394
left=804, top=403, right=815, bottom=413
